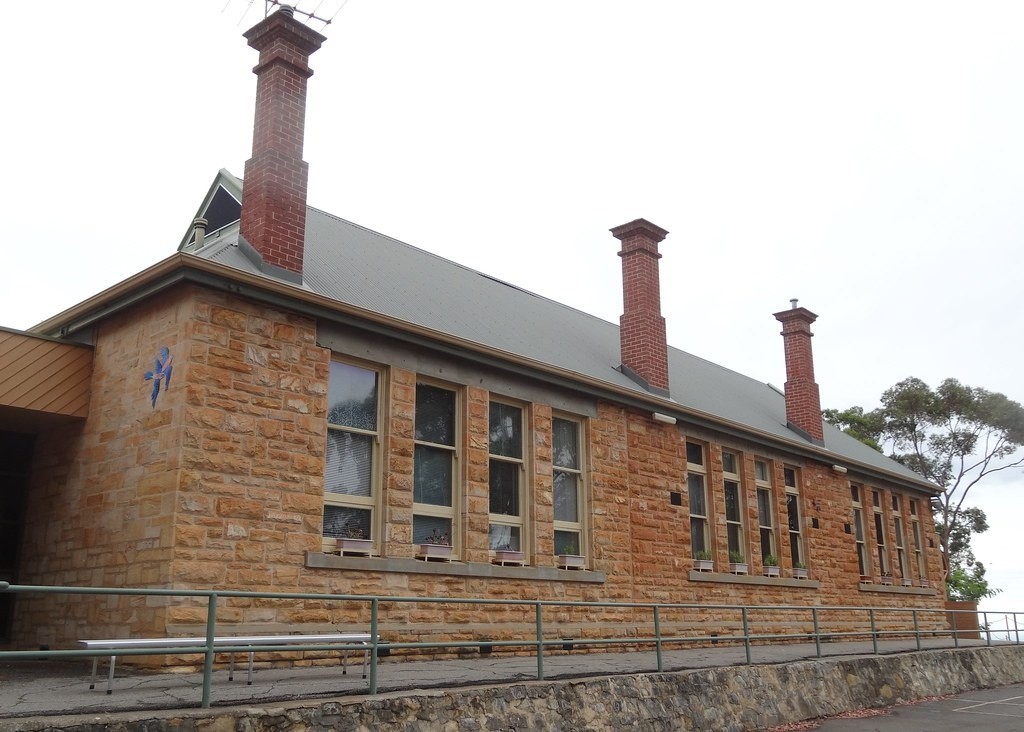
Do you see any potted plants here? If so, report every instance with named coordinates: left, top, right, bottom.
left=559, top=543, right=585, bottom=571
left=919, top=575, right=928, bottom=588
left=793, top=563, right=808, bottom=580
left=420, top=528, right=454, bottom=563
left=729, top=550, right=749, bottom=576
left=693, top=550, right=714, bottom=573
left=496, top=544, right=524, bottom=567
left=763, top=555, right=780, bottom=578
left=335, top=519, right=374, bottom=558
left=881, top=570, right=893, bottom=586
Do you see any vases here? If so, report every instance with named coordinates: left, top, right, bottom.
left=901, top=578, right=912, bottom=587
left=860, top=575, right=873, bottom=584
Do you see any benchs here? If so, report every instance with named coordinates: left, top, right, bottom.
left=77, top=633, right=380, bottom=696
